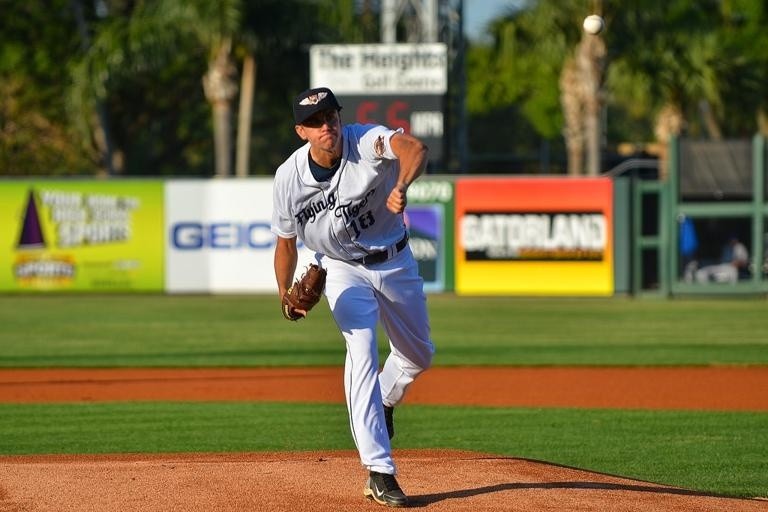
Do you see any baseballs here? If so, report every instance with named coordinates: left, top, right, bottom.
left=582, top=14, right=605, bottom=34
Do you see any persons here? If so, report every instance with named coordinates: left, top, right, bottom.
left=728, top=233, right=751, bottom=278
left=271, top=87, right=437, bottom=508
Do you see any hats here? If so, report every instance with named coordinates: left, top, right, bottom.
left=293, top=88, right=343, bottom=125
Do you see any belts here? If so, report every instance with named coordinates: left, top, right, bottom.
left=353, top=229, right=409, bottom=265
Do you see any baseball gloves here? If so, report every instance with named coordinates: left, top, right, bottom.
left=281, top=266, right=327, bottom=322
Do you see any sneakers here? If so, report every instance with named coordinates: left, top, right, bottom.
left=364, top=470, right=409, bottom=507
left=382, top=403, right=394, bottom=440
